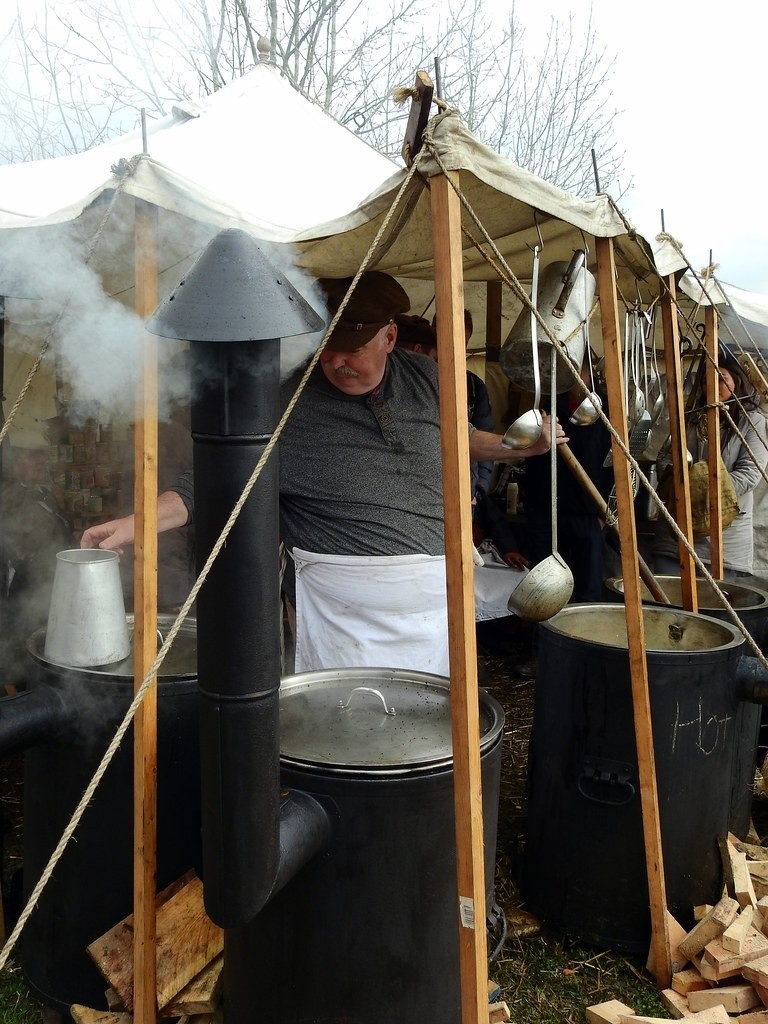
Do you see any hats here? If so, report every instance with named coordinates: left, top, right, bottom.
left=306, top=270, right=410, bottom=352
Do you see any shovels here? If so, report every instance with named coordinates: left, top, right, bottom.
left=628, top=303, right=663, bottom=452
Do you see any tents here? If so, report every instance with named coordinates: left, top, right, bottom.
left=0, top=36, right=768, bottom=1024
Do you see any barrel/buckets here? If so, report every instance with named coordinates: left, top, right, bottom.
left=522, top=573, right=767, bottom=955
left=23, top=611, right=506, bottom=1024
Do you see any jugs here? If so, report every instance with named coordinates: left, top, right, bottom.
left=43, top=548, right=131, bottom=667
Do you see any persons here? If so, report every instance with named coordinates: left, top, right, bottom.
left=652, top=354, right=765, bottom=577
left=80, top=271, right=449, bottom=677
left=394, top=309, right=652, bottom=603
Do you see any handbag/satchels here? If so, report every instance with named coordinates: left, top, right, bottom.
left=673, top=454, right=740, bottom=538
